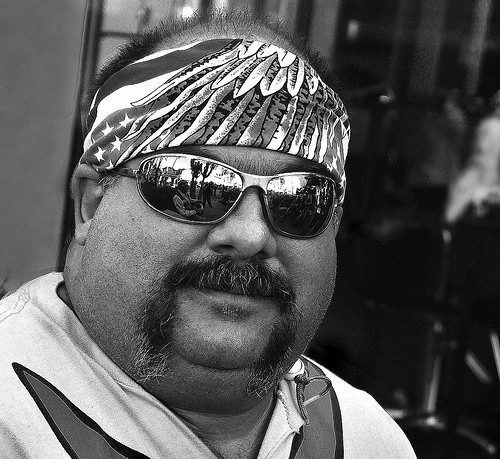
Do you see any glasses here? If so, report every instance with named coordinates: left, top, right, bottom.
left=105, top=153, right=340, bottom=239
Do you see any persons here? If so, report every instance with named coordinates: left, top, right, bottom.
left=398, top=46, right=500, bottom=457
left=0, top=0, right=416, bottom=459
